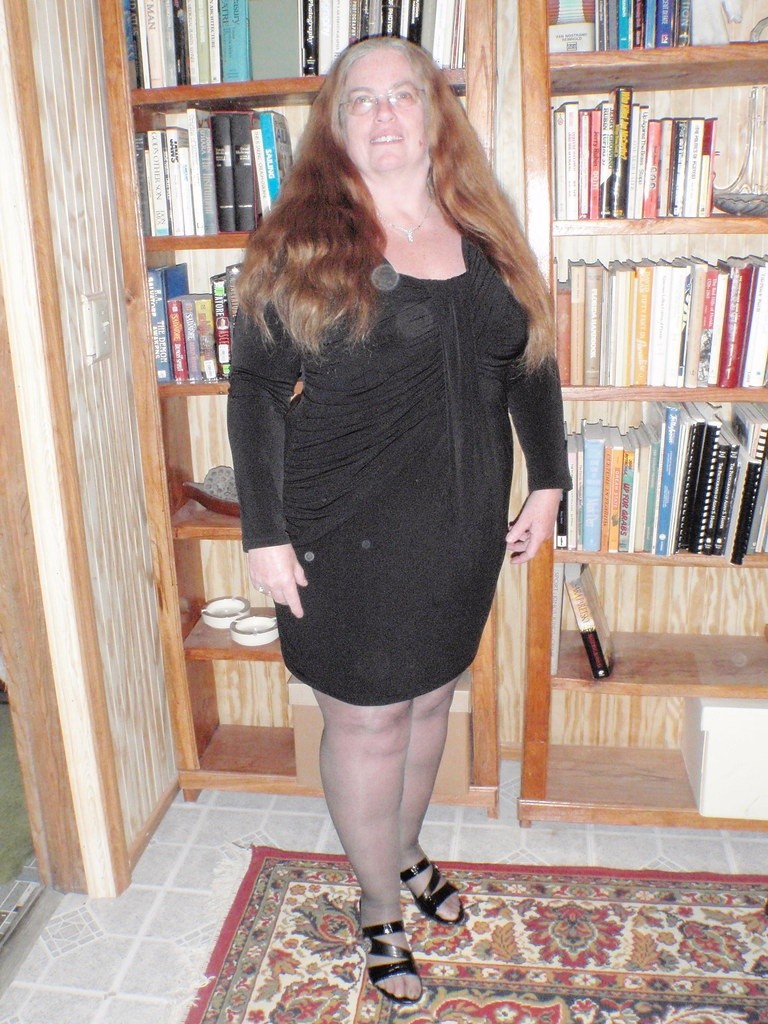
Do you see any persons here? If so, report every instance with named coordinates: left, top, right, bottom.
left=223, top=34, right=572, bottom=1006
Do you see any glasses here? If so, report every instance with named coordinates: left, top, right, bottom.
left=340, top=82, right=424, bottom=115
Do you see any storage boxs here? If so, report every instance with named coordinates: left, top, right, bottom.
left=677, top=695, right=768, bottom=823
left=287, top=672, right=471, bottom=797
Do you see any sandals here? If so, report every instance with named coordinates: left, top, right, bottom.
left=400, top=855, right=465, bottom=925
left=359, top=897, right=423, bottom=1004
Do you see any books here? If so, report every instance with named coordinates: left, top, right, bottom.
left=145, top=257, right=241, bottom=385
left=129, top=104, right=297, bottom=237
left=551, top=250, right=768, bottom=390
left=553, top=400, right=768, bottom=567
left=564, top=563, right=616, bottom=680
left=550, top=87, right=721, bottom=219
left=545, top=0, right=693, bottom=56
left=118, top=0, right=473, bottom=89
left=549, top=563, right=565, bottom=677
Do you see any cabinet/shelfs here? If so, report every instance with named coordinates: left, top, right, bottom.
left=516, top=0, right=768, bottom=831
left=98, top=0, right=501, bottom=823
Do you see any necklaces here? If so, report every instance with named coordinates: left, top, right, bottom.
left=376, top=198, right=433, bottom=243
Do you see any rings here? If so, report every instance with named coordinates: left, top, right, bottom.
left=259, top=587, right=264, bottom=592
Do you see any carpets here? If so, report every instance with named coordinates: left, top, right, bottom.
left=183, top=846, right=768, bottom=1024
